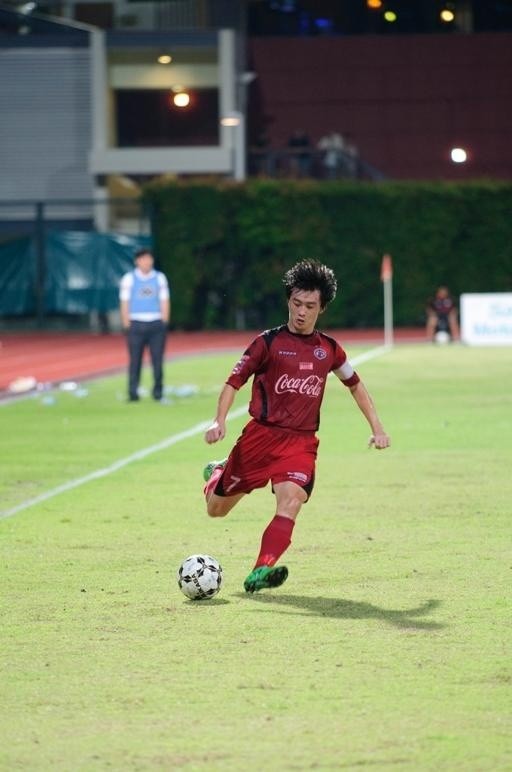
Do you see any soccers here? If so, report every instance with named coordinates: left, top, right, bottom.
left=178, top=555, right=225, bottom=600
left=435, top=331, right=449, bottom=343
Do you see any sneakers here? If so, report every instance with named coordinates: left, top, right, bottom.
left=202, top=458, right=231, bottom=482
left=244, top=564, right=289, bottom=595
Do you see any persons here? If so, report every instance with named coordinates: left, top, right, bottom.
left=199, top=258, right=393, bottom=592
left=251, top=120, right=369, bottom=180
left=424, top=284, right=459, bottom=344
left=118, top=251, right=173, bottom=403
